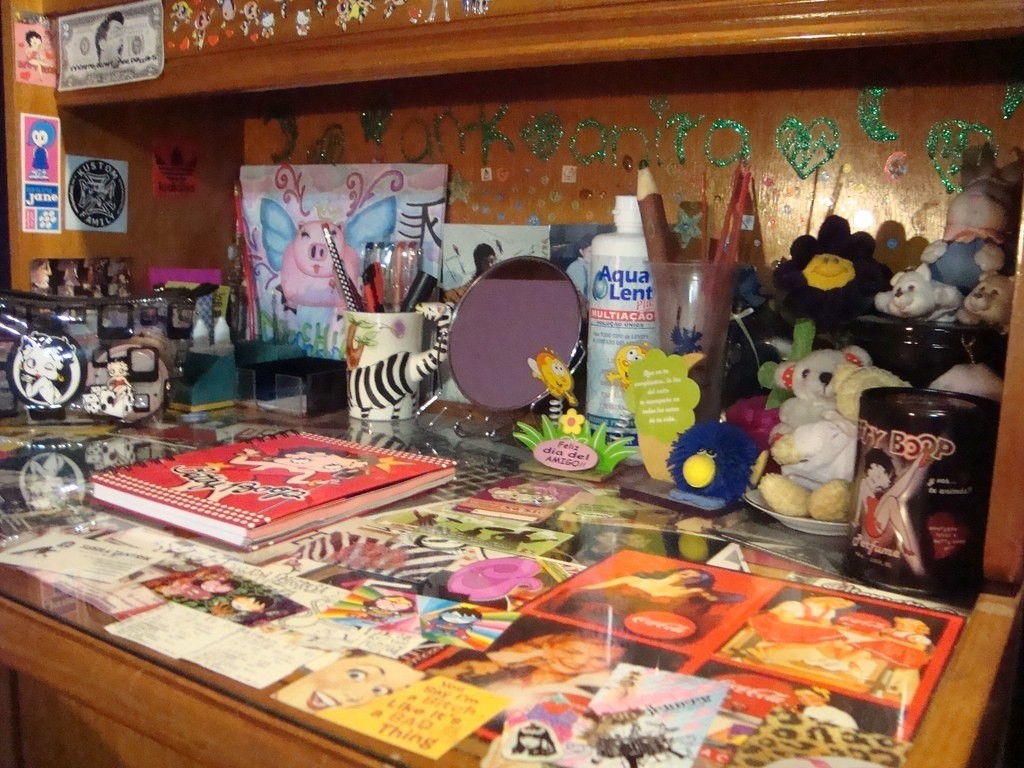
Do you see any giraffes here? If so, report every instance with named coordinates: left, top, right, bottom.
left=345, top=301, right=452, bottom=421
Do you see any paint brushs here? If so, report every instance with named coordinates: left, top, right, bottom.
left=635, top=149, right=752, bottom=262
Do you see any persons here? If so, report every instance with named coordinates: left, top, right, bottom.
left=471, top=242, right=497, bottom=280
left=564, top=235, right=596, bottom=321
left=432, top=559, right=933, bottom=747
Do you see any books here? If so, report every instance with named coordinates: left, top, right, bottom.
left=89, top=423, right=462, bottom=552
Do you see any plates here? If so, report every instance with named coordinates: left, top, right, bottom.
left=741, top=488, right=850, bottom=536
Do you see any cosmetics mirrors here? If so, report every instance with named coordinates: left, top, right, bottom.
left=434, top=256, right=588, bottom=439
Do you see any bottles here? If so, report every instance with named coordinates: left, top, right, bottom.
left=585, top=195, right=667, bottom=466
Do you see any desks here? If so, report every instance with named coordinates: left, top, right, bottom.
left=0, top=408, right=1024, bottom=768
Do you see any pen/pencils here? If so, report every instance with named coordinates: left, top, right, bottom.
left=317, top=223, right=440, bottom=312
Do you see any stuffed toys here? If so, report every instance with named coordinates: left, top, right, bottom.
left=666, top=144, right=1024, bottom=528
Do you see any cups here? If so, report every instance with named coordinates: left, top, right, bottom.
left=346, top=304, right=451, bottom=420
left=845, top=386, right=1002, bottom=593
left=642, top=259, right=732, bottom=427
